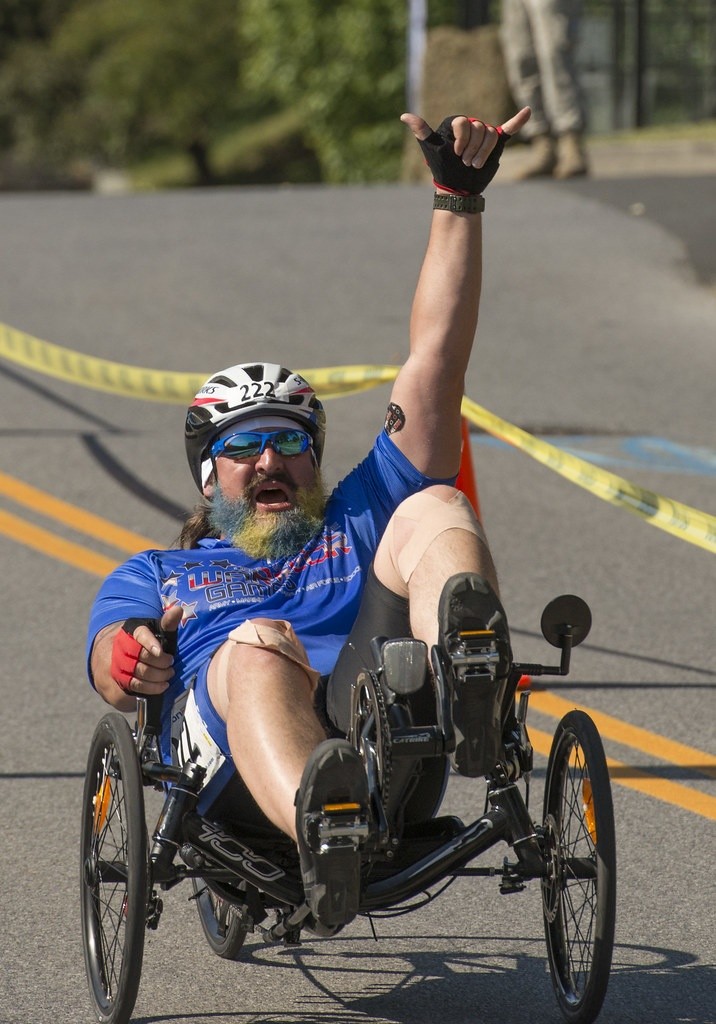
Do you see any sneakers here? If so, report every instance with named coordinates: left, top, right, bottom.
left=439, top=572, right=513, bottom=778
left=296, top=738, right=367, bottom=927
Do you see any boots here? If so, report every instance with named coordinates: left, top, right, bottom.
left=554, top=134, right=589, bottom=179
left=512, top=134, right=556, bottom=180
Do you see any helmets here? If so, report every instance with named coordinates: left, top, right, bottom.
left=184, top=363, right=325, bottom=501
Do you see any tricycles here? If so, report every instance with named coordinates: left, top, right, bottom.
left=79, top=593, right=614, bottom=1024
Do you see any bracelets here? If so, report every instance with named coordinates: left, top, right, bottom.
left=433, top=192, right=486, bottom=213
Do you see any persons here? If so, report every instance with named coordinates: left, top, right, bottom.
left=84, top=107, right=532, bottom=934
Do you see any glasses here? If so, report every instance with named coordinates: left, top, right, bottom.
left=206, top=429, right=313, bottom=459
